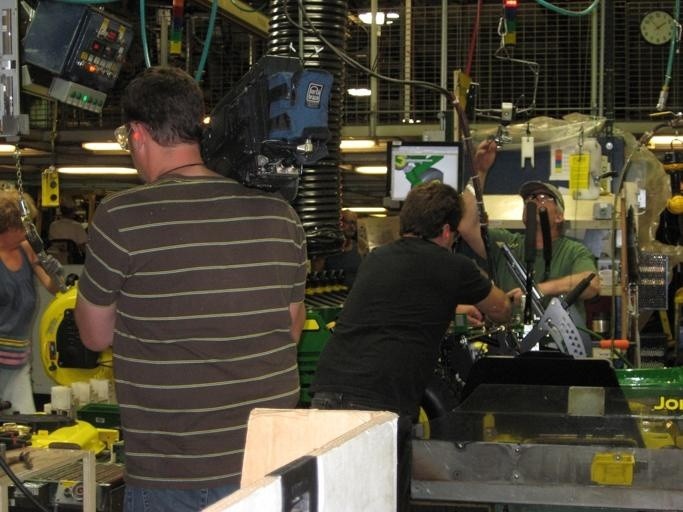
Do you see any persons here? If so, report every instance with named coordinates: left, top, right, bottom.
left=310, top=181, right=515, bottom=512
left=455, top=136, right=599, bottom=327
left=0, top=183, right=62, bottom=413
left=47, top=198, right=89, bottom=248
left=74, top=65, right=313, bottom=511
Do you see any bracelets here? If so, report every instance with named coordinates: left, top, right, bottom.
left=466, top=184, right=480, bottom=198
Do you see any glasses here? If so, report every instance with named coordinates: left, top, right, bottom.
left=523, top=192, right=556, bottom=204
left=116, top=122, right=139, bottom=150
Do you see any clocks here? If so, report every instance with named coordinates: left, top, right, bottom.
left=638, top=9, right=674, bottom=46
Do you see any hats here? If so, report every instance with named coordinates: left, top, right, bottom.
left=519, top=180, right=563, bottom=209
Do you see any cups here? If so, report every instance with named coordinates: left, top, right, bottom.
left=53, top=388, right=72, bottom=411
left=91, top=380, right=110, bottom=403
left=73, top=383, right=91, bottom=407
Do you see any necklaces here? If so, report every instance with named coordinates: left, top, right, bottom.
left=157, top=163, right=203, bottom=177
